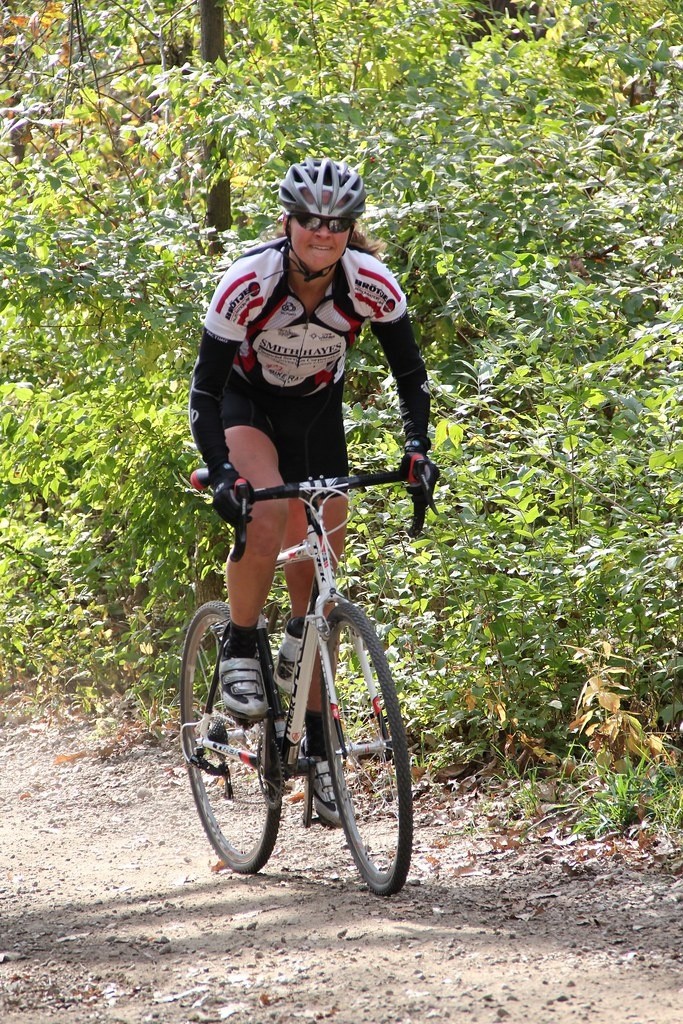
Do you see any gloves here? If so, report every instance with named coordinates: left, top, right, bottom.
left=212, top=476, right=256, bottom=526
left=400, top=452, right=440, bottom=490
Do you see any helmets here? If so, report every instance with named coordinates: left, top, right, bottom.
left=279, top=158, right=366, bottom=219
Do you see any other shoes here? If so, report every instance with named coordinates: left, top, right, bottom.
left=219, top=639, right=269, bottom=720
left=311, top=759, right=355, bottom=828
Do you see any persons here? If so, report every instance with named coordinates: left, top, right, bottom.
left=188, top=155, right=440, bottom=828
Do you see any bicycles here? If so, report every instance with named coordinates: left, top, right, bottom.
left=176, top=447, right=440, bottom=898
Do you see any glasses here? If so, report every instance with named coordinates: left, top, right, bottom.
left=287, top=212, right=355, bottom=234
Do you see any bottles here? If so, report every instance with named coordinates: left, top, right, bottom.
left=273, top=617, right=305, bottom=690
